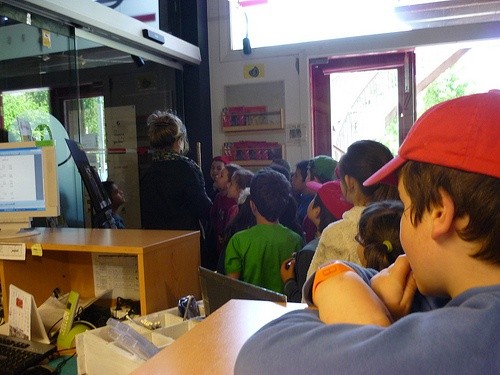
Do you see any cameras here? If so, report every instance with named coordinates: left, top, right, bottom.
left=286, top=251, right=296, bottom=270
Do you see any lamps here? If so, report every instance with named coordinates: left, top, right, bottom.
left=132, top=54, right=145, bottom=69
left=243, top=11, right=251, bottom=55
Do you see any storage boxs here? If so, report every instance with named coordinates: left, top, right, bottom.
left=75, top=300, right=207, bottom=375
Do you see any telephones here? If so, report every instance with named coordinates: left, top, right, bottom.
left=57, top=290, right=89, bottom=349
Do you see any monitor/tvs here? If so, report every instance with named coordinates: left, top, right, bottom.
left=0, top=142, right=61, bottom=238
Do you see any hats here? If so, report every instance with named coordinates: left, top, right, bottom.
left=211, top=156, right=228, bottom=164
left=362, top=89, right=500, bottom=187
left=307, top=155, right=339, bottom=180
left=306, top=181, right=353, bottom=220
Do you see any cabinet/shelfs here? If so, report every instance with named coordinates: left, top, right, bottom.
left=223, top=107, right=286, bottom=166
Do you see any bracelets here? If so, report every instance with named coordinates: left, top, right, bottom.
left=308, top=264, right=356, bottom=311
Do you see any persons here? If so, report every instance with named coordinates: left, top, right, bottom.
left=95, top=179, right=126, bottom=231
left=206, top=140, right=405, bottom=302
left=138, top=109, right=206, bottom=240
left=234, top=89, right=500, bottom=375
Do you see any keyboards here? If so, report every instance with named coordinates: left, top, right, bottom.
left=0, top=334, right=56, bottom=375
